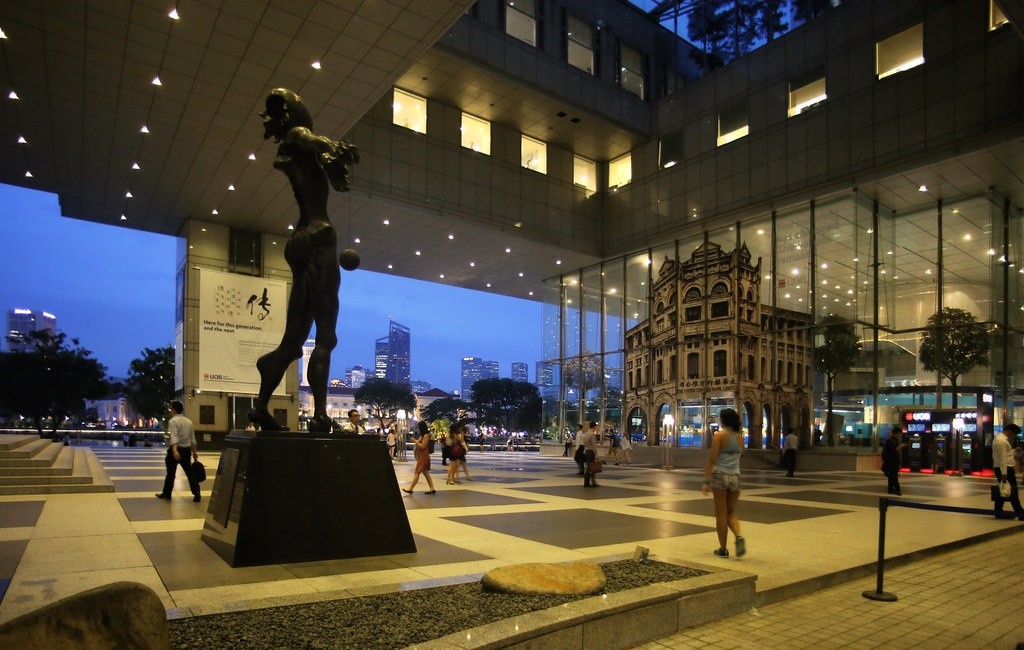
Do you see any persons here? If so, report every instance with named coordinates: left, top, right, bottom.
left=993, top=423, right=1024, bottom=520
left=442, top=424, right=473, bottom=484
left=402, top=421, right=436, bottom=494
left=479, top=430, right=514, bottom=451
left=344, top=409, right=364, bottom=433
left=563, top=434, right=572, bottom=456
left=414, top=427, right=445, bottom=440
left=574, top=422, right=599, bottom=487
left=155, top=401, right=201, bottom=502
left=782, top=430, right=798, bottom=477
left=703, top=409, right=746, bottom=558
left=246, top=87, right=359, bottom=433
left=604, top=429, right=631, bottom=465
left=882, top=427, right=905, bottom=495
left=376, top=428, right=396, bottom=457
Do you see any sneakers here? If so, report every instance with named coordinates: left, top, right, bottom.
left=714, top=548, right=729, bottom=557
left=736, top=536, right=745, bottom=557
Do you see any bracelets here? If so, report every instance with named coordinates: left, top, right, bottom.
left=704, top=480, right=711, bottom=484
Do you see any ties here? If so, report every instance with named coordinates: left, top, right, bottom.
left=355, top=427, right=358, bottom=431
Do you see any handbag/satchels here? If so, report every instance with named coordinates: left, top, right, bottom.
left=452, top=443, right=464, bottom=454
left=1000, top=479, right=1011, bottom=497
left=428, top=440, right=435, bottom=453
left=192, top=459, right=206, bottom=482
left=991, top=483, right=1001, bottom=501
left=588, top=458, right=605, bottom=474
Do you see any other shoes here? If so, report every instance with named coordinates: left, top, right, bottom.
left=403, top=489, right=413, bottom=494
left=425, top=490, right=435, bottom=494
left=193, top=495, right=201, bottom=503
left=614, top=463, right=619, bottom=465
left=156, top=494, right=171, bottom=500
left=601, top=460, right=607, bottom=464
